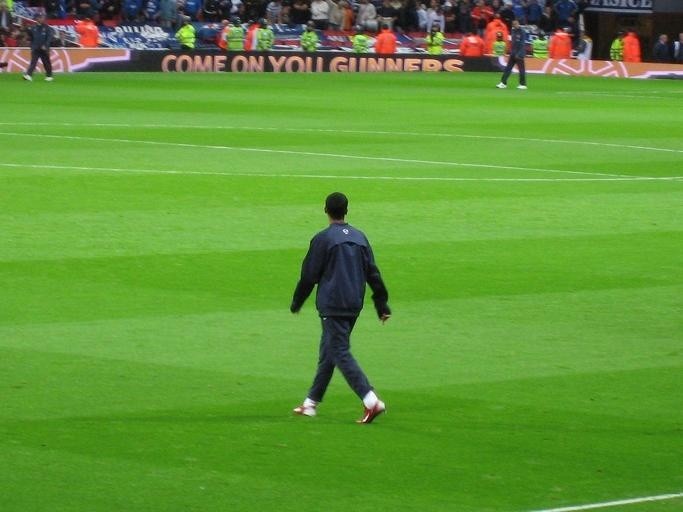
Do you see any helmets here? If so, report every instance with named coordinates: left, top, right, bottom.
left=183, top=16, right=441, bottom=31
left=496, top=32, right=546, bottom=40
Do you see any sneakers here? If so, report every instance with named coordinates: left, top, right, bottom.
left=354, top=400, right=385, bottom=424
left=495, top=82, right=506, bottom=88
left=44, top=77, right=52, bottom=81
left=516, top=84, right=526, bottom=89
left=293, top=405, right=315, bottom=417
left=23, top=74, right=32, bottom=80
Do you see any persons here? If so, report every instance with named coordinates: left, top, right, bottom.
left=1, top=0, right=682, bottom=89
left=289, top=192, right=392, bottom=424
